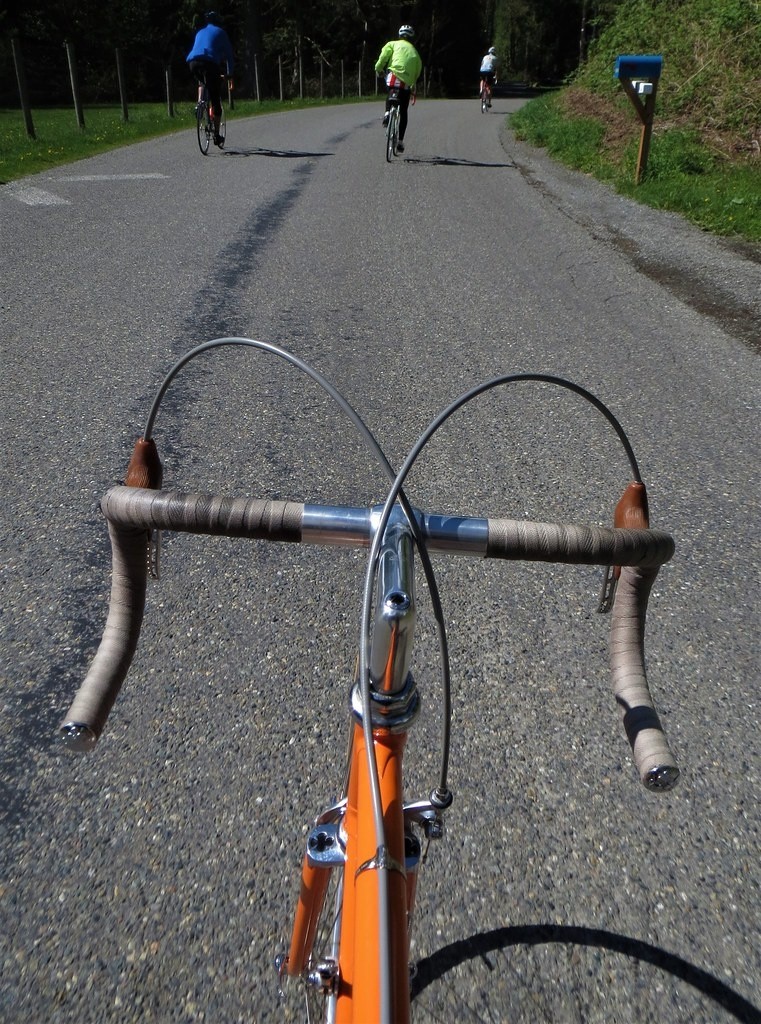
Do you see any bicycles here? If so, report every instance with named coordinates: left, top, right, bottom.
left=385, top=91, right=416, bottom=162
left=481, top=75, right=498, bottom=114
left=195, top=74, right=233, bottom=154
left=52, top=337, right=683, bottom=1024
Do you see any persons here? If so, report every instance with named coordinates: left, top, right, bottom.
left=375, top=25, right=421, bottom=152
left=479, top=47, right=498, bottom=108
left=186, top=11, right=234, bottom=145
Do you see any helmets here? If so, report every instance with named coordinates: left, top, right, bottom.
left=487, top=47, right=496, bottom=54
left=397, top=25, right=415, bottom=37
left=206, top=11, right=218, bottom=19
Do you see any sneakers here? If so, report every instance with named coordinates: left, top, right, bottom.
left=396, top=143, right=404, bottom=154
left=488, top=102, right=492, bottom=108
left=195, top=102, right=204, bottom=119
left=212, top=135, right=225, bottom=145
left=382, top=115, right=390, bottom=125
